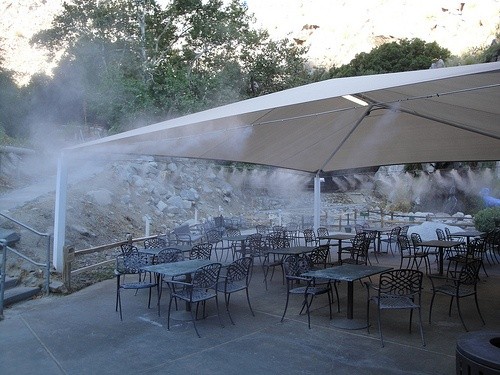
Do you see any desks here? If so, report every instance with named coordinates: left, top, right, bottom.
left=269, top=246, right=322, bottom=289
left=136, top=246, right=198, bottom=257
left=300, top=263, right=394, bottom=329
left=362, top=228, right=391, bottom=253
left=139, top=259, right=234, bottom=321
left=317, top=234, right=356, bottom=265
left=221, top=235, right=256, bottom=268
left=449, top=231, right=484, bottom=259
left=414, top=240, right=463, bottom=280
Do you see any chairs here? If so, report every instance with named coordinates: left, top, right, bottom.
left=116, top=216, right=500, bottom=347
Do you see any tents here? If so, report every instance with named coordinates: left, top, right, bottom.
left=53, top=62, right=500, bottom=270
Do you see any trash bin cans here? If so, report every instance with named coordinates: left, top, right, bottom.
left=456, top=329, right=500, bottom=375
